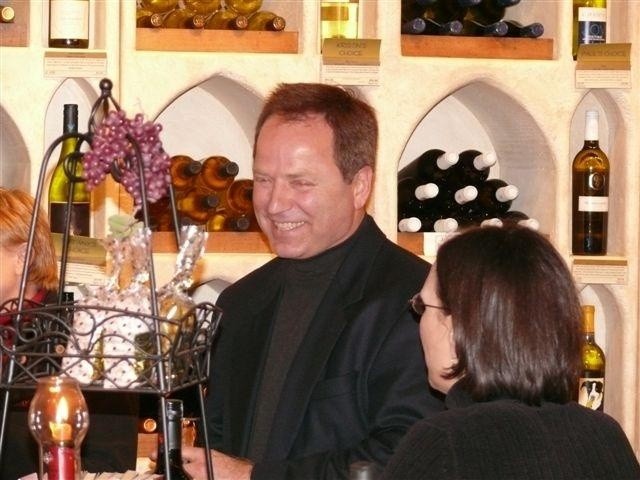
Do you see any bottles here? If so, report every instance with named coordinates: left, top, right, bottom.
left=46, top=104, right=90, bottom=239
left=321, top=0, right=358, bottom=55
left=147, top=399, right=193, bottom=480
left=573, top=0, right=607, bottom=62
left=396, top=149, right=540, bottom=233
left=575, top=306, right=605, bottom=413
left=60, top=222, right=208, bottom=390
left=137, top=0, right=285, bottom=32
left=48, top=0, right=90, bottom=50
left=573, top=108, right=610, bottom=257
left=133, top=155, right=261, bottom=233
left=401, top=0, right=544, bottom=39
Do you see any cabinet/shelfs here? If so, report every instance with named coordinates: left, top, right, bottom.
left=0, top=0, right=640, bottom=446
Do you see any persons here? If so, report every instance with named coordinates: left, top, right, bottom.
left=150, top=82, right=455, bottom=480
left=1, top=187, right=140, bottom=479
left=377, top=224, right=640, bottom=479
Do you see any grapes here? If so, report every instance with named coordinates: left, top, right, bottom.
left=83, top=109, right=173, bottom=207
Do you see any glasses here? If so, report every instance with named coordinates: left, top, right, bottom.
left=408, top=294, right=444, bottom=315
